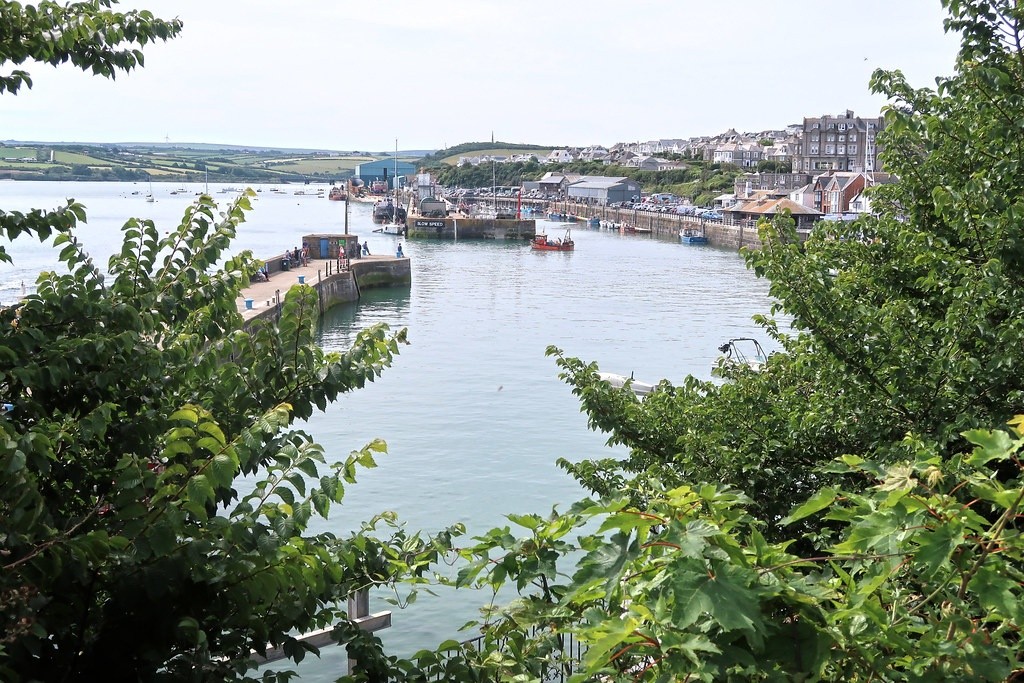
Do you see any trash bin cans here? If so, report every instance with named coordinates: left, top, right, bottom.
left=298, top=276, right=305, bottom=284
left=244, top=299, right=254, bottom=309
left=282, top=259, right=289, bottom=271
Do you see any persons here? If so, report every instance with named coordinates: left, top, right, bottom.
left=338, top=244, right=344, bottom=260
left=364, top=241, right=371, bottom=255
left=256, top=262, right=272, bottom=281
left=285, top=239, right=310, bottom=266
left=398, top=243, right=406, bottom=258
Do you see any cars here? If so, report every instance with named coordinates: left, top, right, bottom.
left=479, top=189, right=561, bottom=202
left=612, top=201, right=723, bottom=222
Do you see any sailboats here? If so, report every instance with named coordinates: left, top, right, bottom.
left=146, top=176, right=154, bottom=202
left=383, top=139, right=402, bottom=235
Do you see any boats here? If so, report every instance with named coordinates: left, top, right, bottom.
left=328, top=187, right=347, bottom=201
left=587, top=215, right=635, bottom=234
left=678, top=225, right=708, bottom=244
left=529, top=228, right=575, bottom=250
left=373, top=198, right=407, bottom=224
